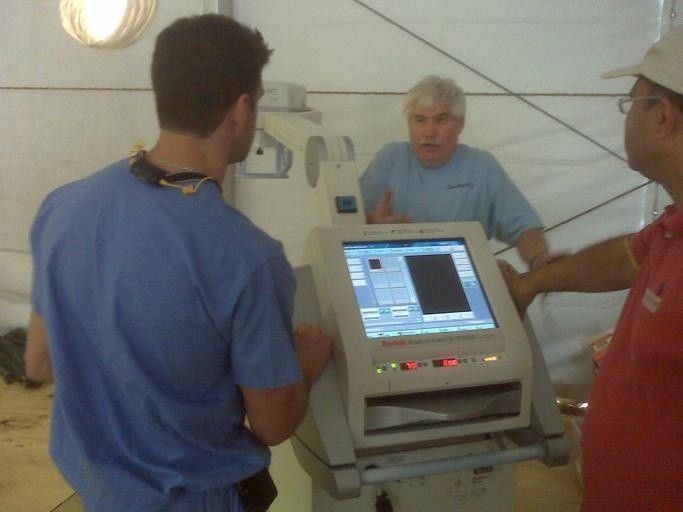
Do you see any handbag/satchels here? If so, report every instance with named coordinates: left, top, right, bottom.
left=0, top=328, right=43, bottom=389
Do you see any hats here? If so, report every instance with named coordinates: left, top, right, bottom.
left=601, top=25, right=682, bottom=96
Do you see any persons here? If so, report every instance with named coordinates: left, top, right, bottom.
left=355, top=72, right=571, bottom=276
left=18, top=9, right=333, bottom=512
left=494, top=21, right=682, bottom=512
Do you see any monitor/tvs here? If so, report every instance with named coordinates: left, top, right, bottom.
left=341, top=236, right=499, bottom=340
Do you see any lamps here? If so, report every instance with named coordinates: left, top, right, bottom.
left=59, top=1, right=157, bottom=48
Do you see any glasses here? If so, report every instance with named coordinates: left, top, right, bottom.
left=617, top=95, right=660, bottom=114
